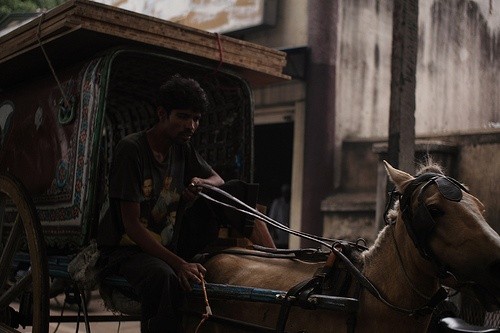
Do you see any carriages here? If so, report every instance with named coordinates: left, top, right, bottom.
left=0, top=0, right=500, bottom=333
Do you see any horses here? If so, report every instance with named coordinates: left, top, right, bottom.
left=142, top=159, right=499, bottom=333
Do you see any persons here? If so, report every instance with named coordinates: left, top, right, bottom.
left=95, top=77, right=278, bottom=333
left=119, top=175, right=179, bottom=247
